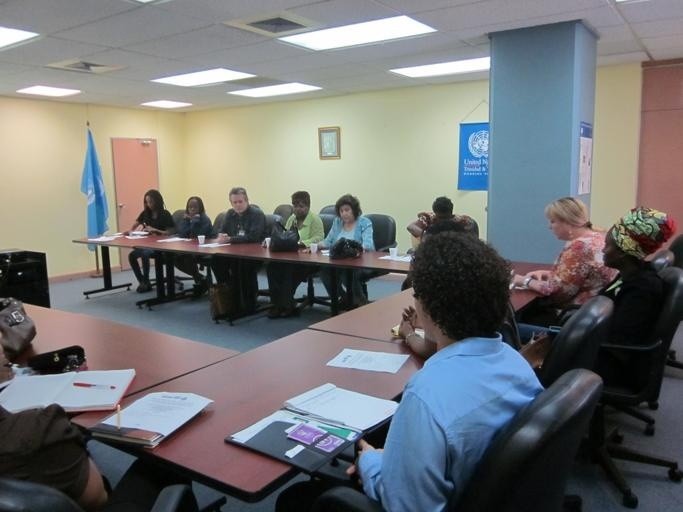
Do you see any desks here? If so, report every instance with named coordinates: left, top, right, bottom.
left=2, top=298, right=241, bottom=422
left=72, top=232, right=411, bottom=326
left=309, top=259, right=558, bottom=349
left=69, top=328, right=442, bottom=502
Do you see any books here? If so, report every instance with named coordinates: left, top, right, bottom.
left=86, top=391, right=215, bottom=449
left=0, top=367, right=137, bottom=413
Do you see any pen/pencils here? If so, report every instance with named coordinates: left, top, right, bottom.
left=180, top=237, right=189, bottom=239
left=74, top=380, right=117, bottom=393
left=115, top=404, right=125, bottom=434
left=144, top=223, right=146, bottom=229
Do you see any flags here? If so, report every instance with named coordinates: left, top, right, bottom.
left=80, top=120, right=109, bottom=278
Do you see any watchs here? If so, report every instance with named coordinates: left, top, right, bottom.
left=522, top=276, right=532, bottom=290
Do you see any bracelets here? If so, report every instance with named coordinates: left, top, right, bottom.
left=405, top=332, right=420, bottom=347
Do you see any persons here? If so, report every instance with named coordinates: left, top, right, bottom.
left=534, top=206, right=677, bottom=429
left=266, top=191, right=325, bottom=319
left=302, top=194, right=376, bottom=308
left=357, top=230, right=546, bottom=511
left=401, top=196, right=474, bottom=291
left=123, top=189, right=175, bottom=293
left=211, top=188, right=265, bottom=309
left=174, top=197, right=213, bottom=300
left=510, top=196, right=618, bottom=315
left=0, top=403, right=109, bottom=512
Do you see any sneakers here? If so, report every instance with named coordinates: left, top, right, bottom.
left=266, top=305, right=279, bottom=318
left=278, top=307, right=299, bottom=318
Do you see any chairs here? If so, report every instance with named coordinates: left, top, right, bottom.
left=529, top=296, right=682, bottom=509
left=186, top=211, right=228, bottom=300
left=289, top=213, right=340, bottom=315
left=321, top=205, right=340, bottom=215
left=447, top=214, right=478, bottom=236
left=310, top=365, right=606, bottom=510
left=273, top=204, right=293, bottom=227
left=361, top=213, right=398, bottom=310
left=145, top=208, right=196, bottom=289
left=234, top=204, right=271, bottom=309
left=556, top=247, right=678, bottom=410
left=661, top=229, right=682, bottom=375
left=547, top=266, right=683, bottom=438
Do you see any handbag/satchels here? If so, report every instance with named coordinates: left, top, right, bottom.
left=27, top=345, right=85, bottom=374
left=0, top=297, right=37, bottom=351
left=329, top=237, right=363, bottom=259
left=268, top=219, right=299, bottom=253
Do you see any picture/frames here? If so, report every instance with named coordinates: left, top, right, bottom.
left=317, top=126, right=342, bottom=160
